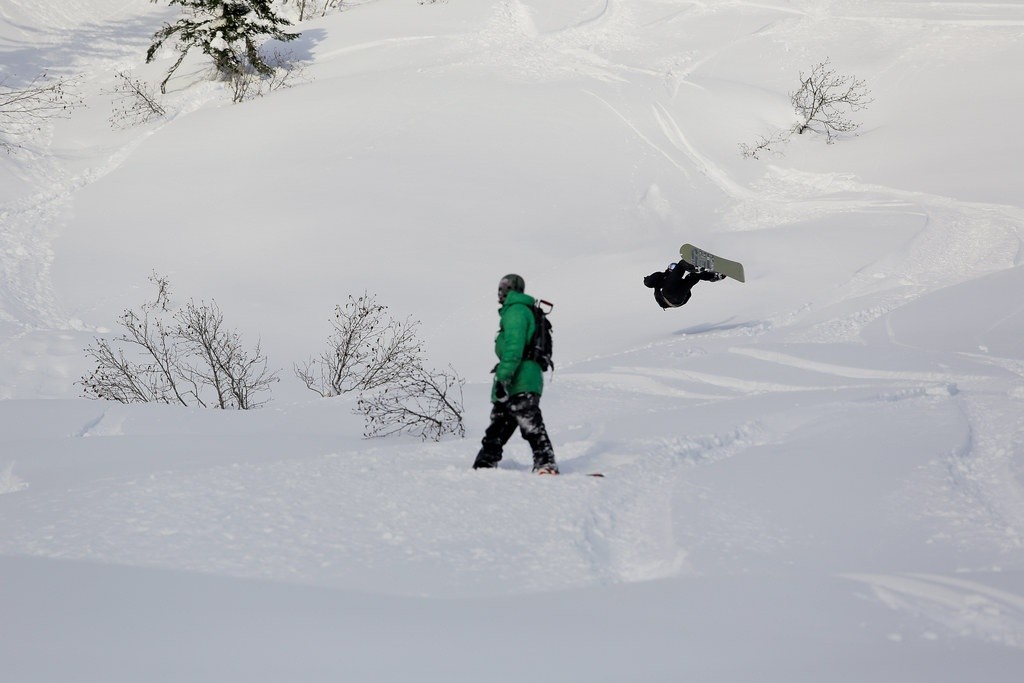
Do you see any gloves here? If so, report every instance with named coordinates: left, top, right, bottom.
left=495, top=380, right=510, bottom=404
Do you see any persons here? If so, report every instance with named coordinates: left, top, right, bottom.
left=644, top=259, right=726, bottom=309
left=472, top=273, right=560, bottom=476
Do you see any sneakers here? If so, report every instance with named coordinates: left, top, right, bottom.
left=532, top=463, right=560, bottom=475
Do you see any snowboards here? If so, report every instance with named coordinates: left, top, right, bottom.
left=587, top=473, right=605, bottom=478
left=680, top=244, right=745, bottom=283
